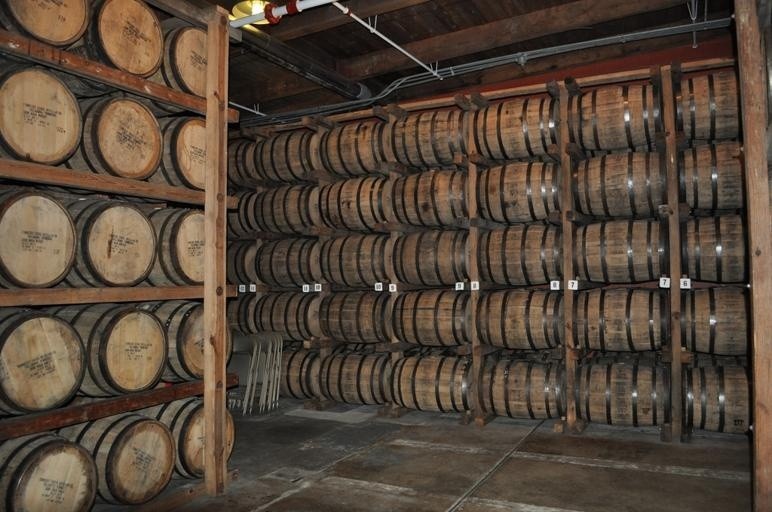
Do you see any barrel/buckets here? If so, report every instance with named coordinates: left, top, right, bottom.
left=252, top=237, right=328, bottom=286
left=0, top=430, right=99, bottom=512
left=227, top=240, right=262, bottom=284
left=253, top=286, right=323, bottom=341
left=226, top=290, right=258, bottom=335
left=227, top=133, right=258, bottom=184
left=0, top=0, right=91, bottom=49
left=469, top=92, right=561, bottom=162
left=682, top=285, right=754, bottom=355
left=56, top=197, right=157, bottom=287
left=478, top=221, right=563, bottom=288
left=670, top=65, right=742, bottom=137
left=318, top=175, right=394, bottom=232
left=0, top=305, right=86, bottom=417
left=318, top=290, right=394, bottom=343
left=319, top=118, right=391, bottom=174
left=319, top=353, right=391, bottom=406
left=146, top=116, right=207, bottom=191
left=54, top=412, right=176, bottom=505
left=393, top=226, right=472, bottom=286
left=141, top=206, right=205, bottom=286
left=388, top=353, right=473, bottom=413
left=571, top=150, right=667, bottom=219
left=138, top=298, right=234, bottom=385
left=575, top=358, right=673, bottom=425
left=0, top=187, right=79, bottom=289
left=320, top=231, right=394, bottom=290
left=476, top=353, right=566, bottom=419
left=253, top=183, right=321, bottom=233
left=675, top=138, right=746, bottom=212
left=684, top=365, right=752, bottom=433
left=147, top=25, right=208, bottom=99
left=254, top=127, right=322, bottom=183
left=227, top=189, right=261, bottom=237
left=279, top=346, right=321, bottom=399
left=391, top=287, right=473, bottom=347
left=133, top=394, right=235, bottom=481
left=680, top=213, right=747, bottom=283
left=65, top=0, right=165, bottom=79
left=391, top=107, right=469, bottom=166
left=0, top=63, right=83, bottom=166
left=391, top=167, right=469, bottom=229
left=571, top=287, right=672, bottom=352
left=476, top=159, right=563, bottom=221
left=474, top=287, right=563, bottom=351
left=565, top=79, right=667, bottom=155
left=39, top=304, right=168, bottom=398
left=57, top=93, right=163, bottom=180
left=572, top=217, right=670, bottom=286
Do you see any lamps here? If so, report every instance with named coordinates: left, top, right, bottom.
left=230, top=0, right=282, bottom=25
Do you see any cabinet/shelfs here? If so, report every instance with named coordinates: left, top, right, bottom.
left=228, top=57, right=737, bottom=444
left=1, top=0, right=242, bottom=512
